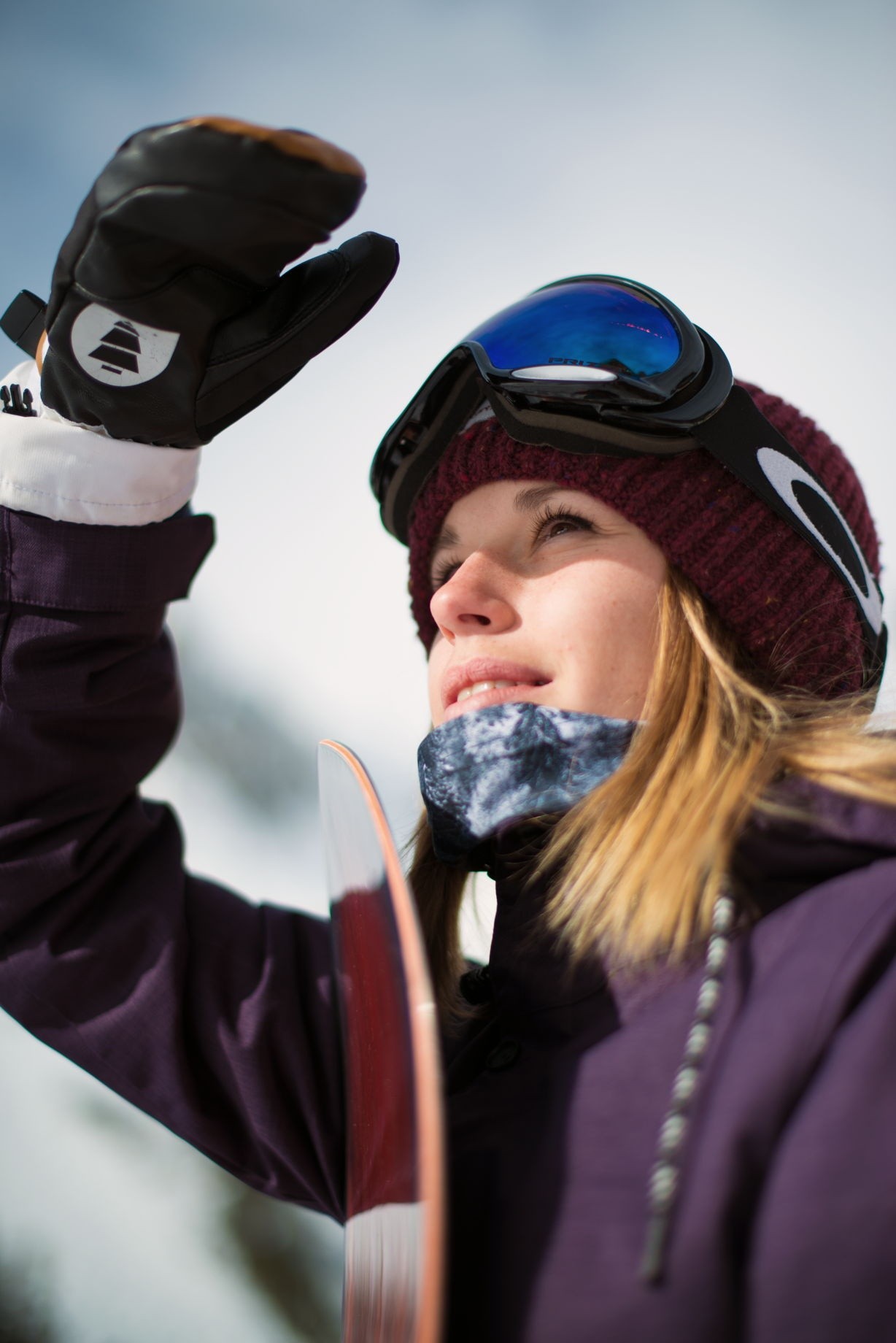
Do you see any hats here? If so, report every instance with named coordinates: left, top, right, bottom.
left=408, top=373, right=885, bottom=715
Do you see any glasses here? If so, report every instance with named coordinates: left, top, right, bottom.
left=369, top=274, right=734, bottom=547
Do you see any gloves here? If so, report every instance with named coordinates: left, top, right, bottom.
left=2, top=119, right=401, bottom=443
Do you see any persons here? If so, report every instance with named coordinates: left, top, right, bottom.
left=0, top=117, right=896, bottom=1343
